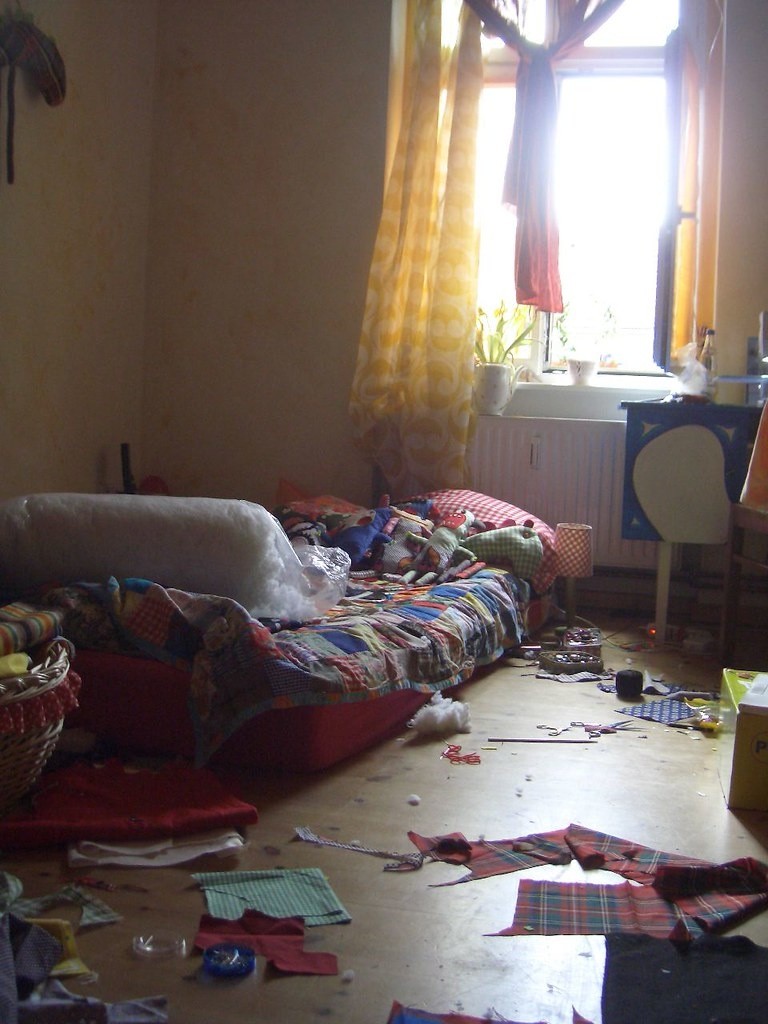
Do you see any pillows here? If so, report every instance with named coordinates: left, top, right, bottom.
left=406, top=490, right=556, bottom=595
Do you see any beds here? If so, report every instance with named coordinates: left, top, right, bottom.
left=1, top=493, right=548, bottom=776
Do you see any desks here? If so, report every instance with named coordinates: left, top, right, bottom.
left=620, top=399, right=763, bottom=641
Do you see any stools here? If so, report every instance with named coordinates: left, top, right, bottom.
left=714, top=504, right=768, bottom=668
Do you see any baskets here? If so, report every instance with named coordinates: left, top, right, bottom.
left=538, top=651, right=604, bottom=674
left=0, top=638, right=81, bottom=805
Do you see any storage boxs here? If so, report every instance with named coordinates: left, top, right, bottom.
left=717, top=668, right=768, bottom=811
left=560, top=627, right=602, bottom=657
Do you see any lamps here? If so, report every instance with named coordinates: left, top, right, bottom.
left=553, top=523, right=592, bottom=633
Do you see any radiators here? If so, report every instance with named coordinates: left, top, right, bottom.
left=472, top=415, right=682, bottom=572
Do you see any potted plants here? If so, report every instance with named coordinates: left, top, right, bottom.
left=473, top=296, right=547, bottom=415
